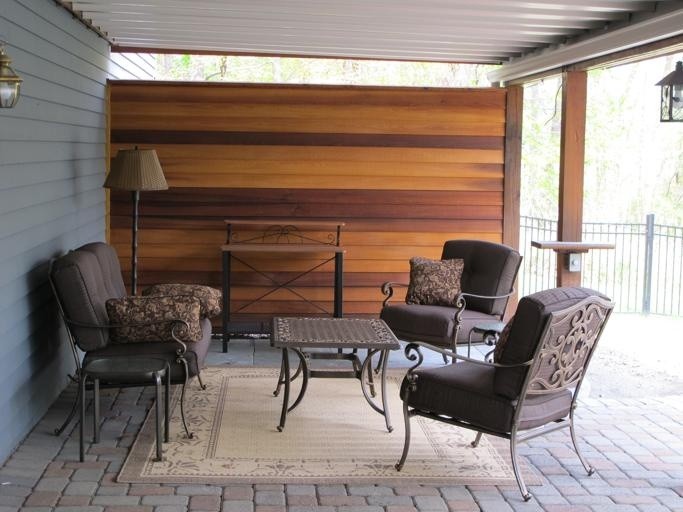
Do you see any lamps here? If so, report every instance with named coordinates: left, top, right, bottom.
left=654, top=48, right=682, bottom=124
left=100, top=141, right=168, bottom=293
left=0, top=40, right=23, bottom=110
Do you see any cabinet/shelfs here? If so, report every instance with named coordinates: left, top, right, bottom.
left=217, top=217, right=347, bottom=354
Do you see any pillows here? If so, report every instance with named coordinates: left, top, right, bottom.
left=406, top=257, right=464, bottom=309
left=152, top=284, right=222, bottom=320
left=103, top=290, right=201, bottom=345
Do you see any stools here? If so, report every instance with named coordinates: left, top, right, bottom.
left=78, top=354, right=172, bottom=464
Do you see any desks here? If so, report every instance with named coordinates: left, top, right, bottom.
left=268, top=316, right=399, bottom=434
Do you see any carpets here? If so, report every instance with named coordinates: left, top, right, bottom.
left=114, top=363, right=546, bottom=487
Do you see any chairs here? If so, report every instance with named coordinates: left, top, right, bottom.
left=373, top=236, right=522, bottom=382
left=51, top=238, right=223, bottom=441
left=393, top=285, right=613, bottom=500
